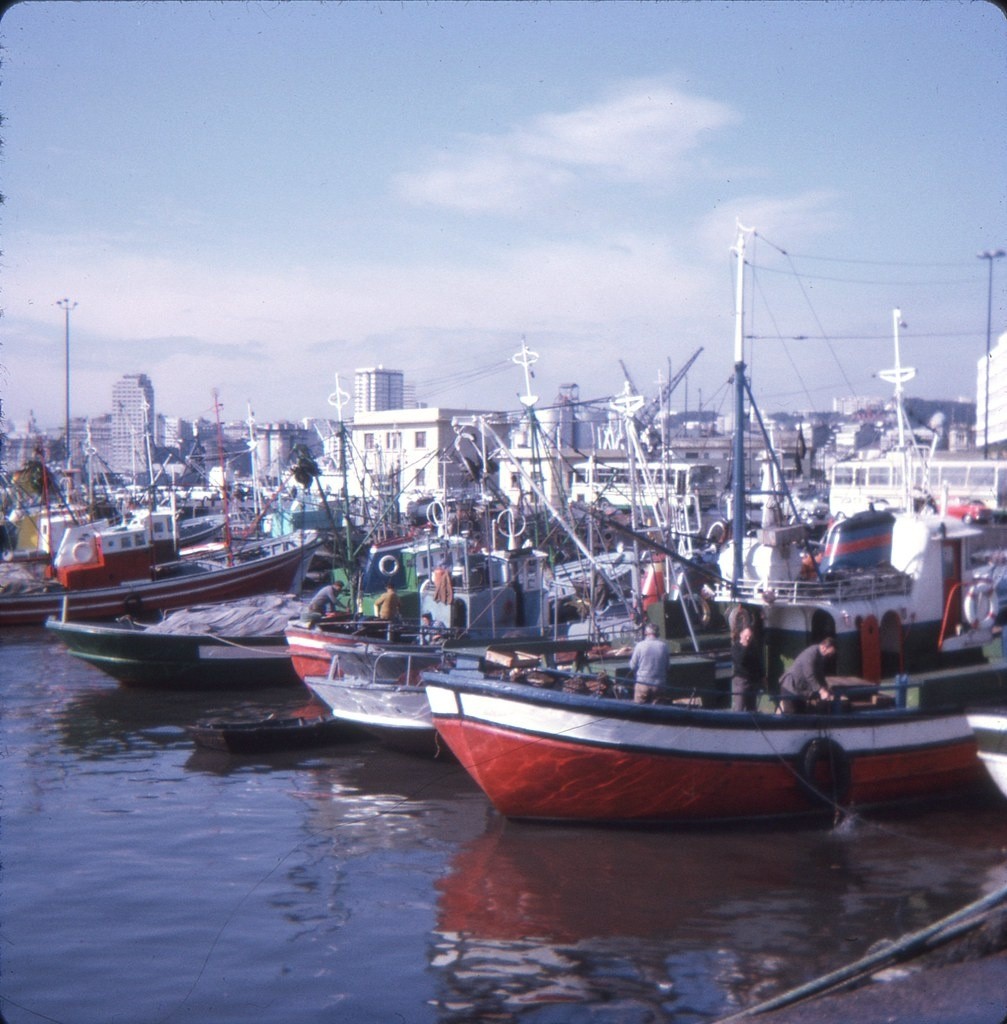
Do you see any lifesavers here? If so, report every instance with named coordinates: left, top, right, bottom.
left=682, top=593, right=711, bottom=633
left=124, top=595, right=142, bottom=614
left=378, top=554, right=400, bottom=577
left=72, top=542, right=95, bottom=565
left=964, top=581, right=1000, bottom=631
left=796, top=736, right=853, bottom=808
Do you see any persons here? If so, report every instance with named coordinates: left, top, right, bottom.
left=308, top=580, right=349, bottom=615
left=630, top=624, right=670, bottom=706
left=731, top=628, right=768, bottom=712
left=775, top=638, right=837, bottom=715
left=416, top=613, right=446, bottom=646
left=376, top=584, right=402, bottom=620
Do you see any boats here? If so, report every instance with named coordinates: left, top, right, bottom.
left=428, top=812, right=1007, bottom=1023
left=287, top=736, right=495, bottom=934
left=0, top=219, right=1007, bottom=821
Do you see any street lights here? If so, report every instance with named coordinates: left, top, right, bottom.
left=975, top=246, right=1007, bottom=460
left=58, top=298, right=79, bottom=460
